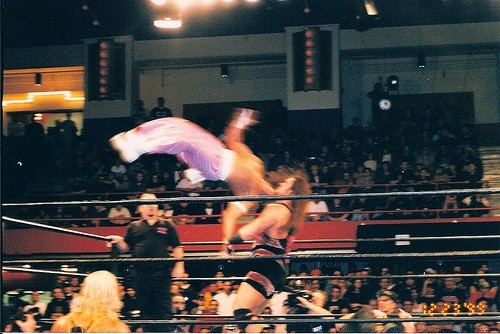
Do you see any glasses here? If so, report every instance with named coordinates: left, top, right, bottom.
left=377, top=298, right=390, bottom=302
left=410, top=291, right=419, bottom=294
left=403, top=303, right=413, bottom=306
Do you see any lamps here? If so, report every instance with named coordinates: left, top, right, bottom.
left=220, top=64, right=228, bottom=78
left=35, top=73, right=42, bottom=86
left=387, top=75, right=398, bottom=95
left=418, top=52, right=425, bottom=68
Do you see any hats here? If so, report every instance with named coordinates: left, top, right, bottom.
left=138, top=193, right=158, bottom=207
left=381, top=291, right=399, bottom=301
left=215, top=272, right=224, bottom=277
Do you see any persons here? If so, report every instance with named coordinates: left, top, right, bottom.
left=0, top=97, right=500, bottom=334
